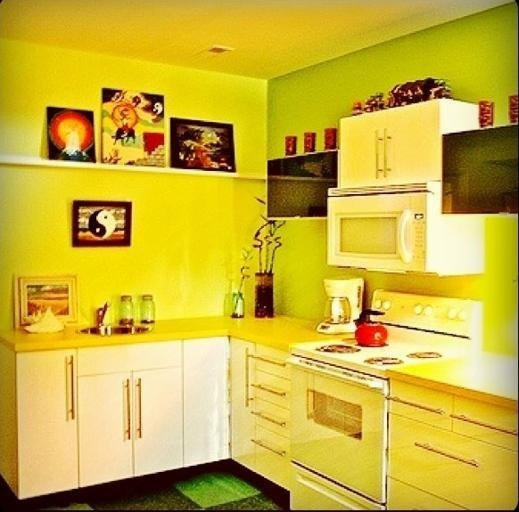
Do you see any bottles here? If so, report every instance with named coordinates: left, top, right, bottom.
left=141, top=295, right=155, bottom=323
left=352, top=101, right=363, bottom=115
left=119, top=296, right=134, bottom=325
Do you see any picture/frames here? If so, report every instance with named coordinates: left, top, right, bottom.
left=170, top=118, right=235, bottom=172
left=14, top=275, right=79, bottom=328
left=72, top=200, right=132, bottom=246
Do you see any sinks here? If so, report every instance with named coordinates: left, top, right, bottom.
left=74, top=324, right=153, bottom=336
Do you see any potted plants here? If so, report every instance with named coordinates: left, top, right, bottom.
left=231, top=249, right=251, bottom=318
left=252, top=198, right=285, bottom=318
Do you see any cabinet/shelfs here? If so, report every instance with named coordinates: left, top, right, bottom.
left=338, top=99, right=480, bottom=186
left=387, top=378, right=519, bottom=511
left=231, top=337, right=290, bottom=511
left=0, top=348, right=79, bottom=499
left=183, top=336, right=230, bottom=486
left=78, top=340, right=183, bottom=503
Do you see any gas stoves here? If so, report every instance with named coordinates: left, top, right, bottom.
left=291, top=326, right=468, bottom=371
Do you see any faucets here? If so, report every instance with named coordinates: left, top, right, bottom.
left=95, top=300, right=112, bottom=328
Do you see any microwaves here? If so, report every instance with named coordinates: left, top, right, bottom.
left=326, top=192, right=485, bottom=273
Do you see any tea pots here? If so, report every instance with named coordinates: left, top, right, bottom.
left=353, top=309, right=387, bottom=346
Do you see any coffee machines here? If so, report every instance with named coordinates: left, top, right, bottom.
left=316, top=276, right=364, bottom=333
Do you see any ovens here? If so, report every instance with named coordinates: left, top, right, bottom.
left=285, top=356, right=388, bottom=510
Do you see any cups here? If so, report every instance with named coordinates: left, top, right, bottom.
left=286, top=136, right=297, bottom=156
left=304, top=133, right=316, bottom=152
left=508, top=95, right=519, bottom=123
left=323, top=128, right=336, bottom=149
left=479, top=101, right=494, bottom=128
